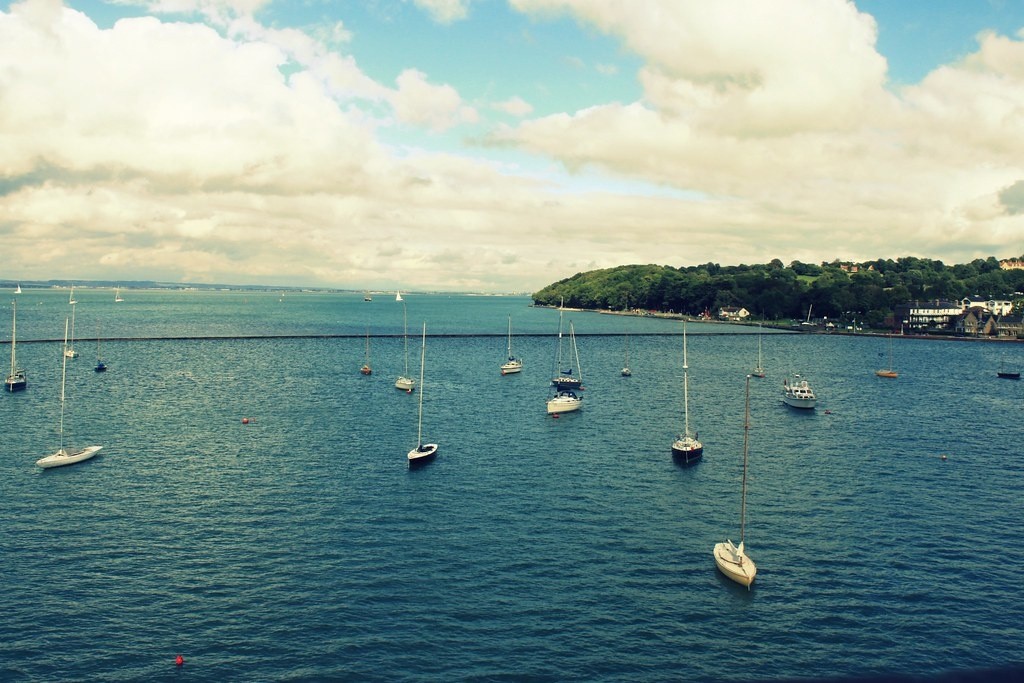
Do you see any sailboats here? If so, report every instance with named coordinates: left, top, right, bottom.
left=115, top=286, right=124, bottom=301
left=14, top=284, right=22, bottom=293
left=94, top=335, right=107, bottom=373
left=546, top=296, right=583, bottom=414
left=66, top=306, right=79, bottom=358
left=875, top=332, right=898, bottom=378
left=396, top=291, right=403, bottom=301
left=4, top=299, right=27, bottom=393
left=712, top=374, right=758, bottom=590
left=751, top=324, right=764, bottom=377
left=364, top=289, right=372, bottom=301
left=69, top=286, right=78, bottom=304
left=360, top=328, right=372, bottom=375
left=501, top=313, right=523, bottom=374
left=671, top=322, right=704, bottom=463
left=35, top=316, right=103, bottom=470
left=551, top=320, right=582, bottom=389
left=406, top=321, right=439, bottom=464
left=395, top=302, right=415, bottom=392
left=621, top=329, right=632, bottom=376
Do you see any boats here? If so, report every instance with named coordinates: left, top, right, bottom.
left=783, top=372, right=817, bottom=409
left=997, top=372, right=1021, bottom=379
